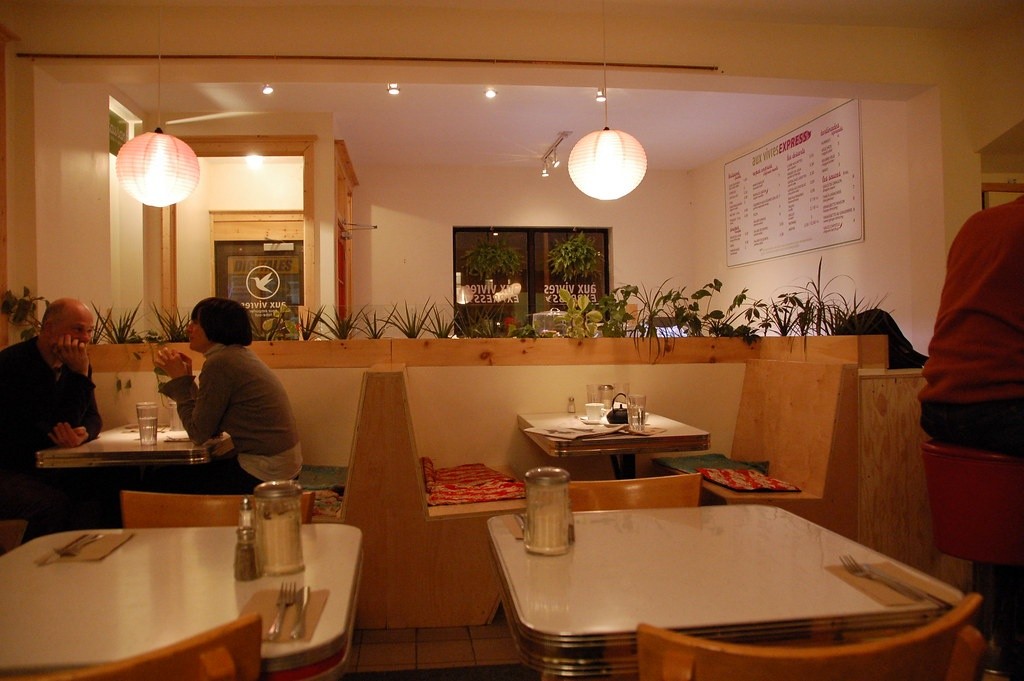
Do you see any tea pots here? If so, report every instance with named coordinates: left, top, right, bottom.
left=606, top=392, right=628, bottom=424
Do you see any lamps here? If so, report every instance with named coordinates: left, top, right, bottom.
left=541, top=160, right=549, bottom=178
left=115, top=0, right=200, bottom=208
left=387, top=83, right=400, bottom=95
left=551, top=147, right=560, bottom=168
left=596, top=88, right=606, bottom=103
left=567, top=0, right=647, bottom=201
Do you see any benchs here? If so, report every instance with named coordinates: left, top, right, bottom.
left=383, top=363, right=525, bottom=628
left=651, top=359, right=858, bottom=542
left=293, top=364, right=383, bottom=631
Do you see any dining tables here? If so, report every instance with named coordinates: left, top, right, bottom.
left=35, top=423, right=212, bottom=492
left=518, top=412, right=711, bottom=480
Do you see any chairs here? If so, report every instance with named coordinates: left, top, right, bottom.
left=119, top=490, right=315, bottom=529
left=486, top=504, right=966, bottom=681
left=636, top=591, right=987, bottom=681
left=566, top=473, right=702, bottom=511
left=0, top=616, right=262, bottom=681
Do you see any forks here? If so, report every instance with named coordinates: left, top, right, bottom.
left=266, top=580, right=296, bottom=641
left=53, top=534, right=104, bottom=557
left=838, top=554, right=923, bottom=601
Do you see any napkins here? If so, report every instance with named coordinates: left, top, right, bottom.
left=45, top=533, right=133, bottom=562
left=239, top=590, right=329, bottom=642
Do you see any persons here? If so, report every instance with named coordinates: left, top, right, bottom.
left=0, top=297, right=138, bottom=543
left=917, top=193, right=1024, bottom=681
left=144, top=297, right=302, bottom=496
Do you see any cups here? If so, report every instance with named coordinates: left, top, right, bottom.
left=645, top=413, right=649, bottom=424
left=585, top=403, right=607, bottom=421
left=136, top=402, right=158, bottom=446
left=626, top=394, right=646, bottom=433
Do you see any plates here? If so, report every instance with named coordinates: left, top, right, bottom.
left=581, top=419, right=608, bottom=425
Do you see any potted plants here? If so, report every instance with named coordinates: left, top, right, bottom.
left=545, top=232, right=605, bottom=283
left=461, top=234, right=522, bottom=280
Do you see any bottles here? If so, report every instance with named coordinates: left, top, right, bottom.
left=598, top=384, right=614, bottom=409
left=568, top=499, right=575, bottom=544
left=523, top=466, right=571, bottom=555
left=169, top=400, right=185, bottom=431
left=232, top=480, right=306, bottom=581
left=568, top=397, right=575, bottom=413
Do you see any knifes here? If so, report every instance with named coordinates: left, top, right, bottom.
left=46, top=534, right=91, bottom=564
left=869, top=566, right=952, bottom=608
left=289, top=586, right=310, bottom=639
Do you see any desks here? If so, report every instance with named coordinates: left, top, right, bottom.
left=0, top=523, right=364, bottom=681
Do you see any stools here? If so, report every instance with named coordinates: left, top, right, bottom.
left=920, top=438, right=1024, bottom=675
